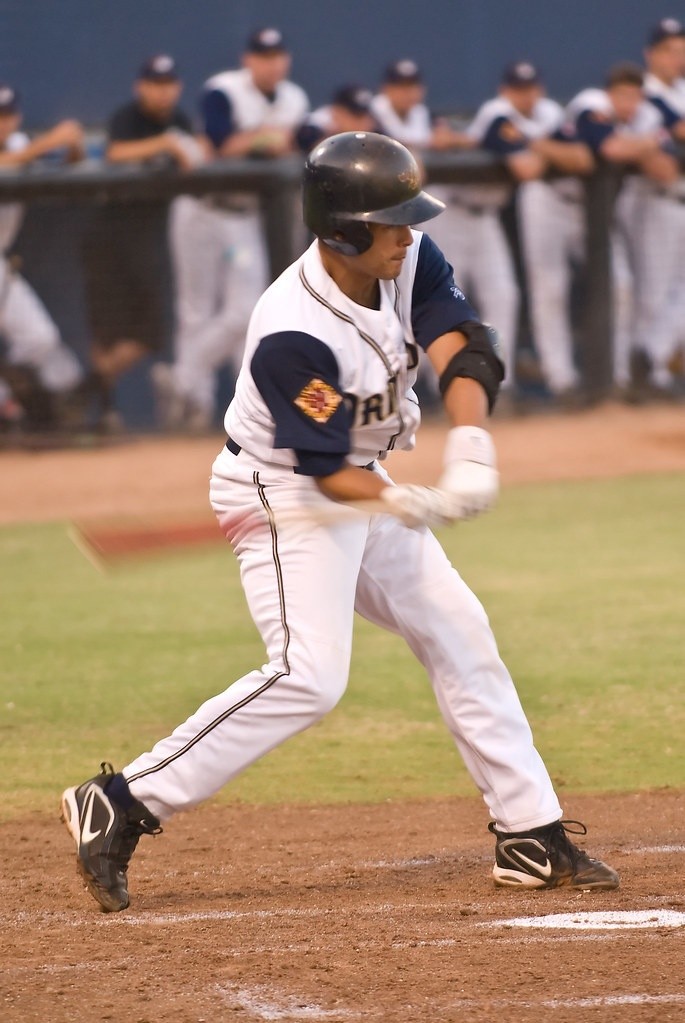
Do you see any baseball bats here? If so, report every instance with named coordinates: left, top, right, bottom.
left=64, top=499, right=400, bottom=572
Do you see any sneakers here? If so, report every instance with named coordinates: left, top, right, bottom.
left=487, top=821, right=620, bottom=890
left=55, top=761, right=163, bottom=912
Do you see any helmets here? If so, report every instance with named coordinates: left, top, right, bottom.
left=301, top=129, right=447, bottom=258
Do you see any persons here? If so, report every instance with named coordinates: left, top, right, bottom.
left=61, top=131, right=621, bottom=914
left=1, top=16, right=684, bottom=447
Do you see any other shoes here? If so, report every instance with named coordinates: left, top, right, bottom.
left=50, top=386, right=105, bottom=432
left=86, top=341, right=119, bottom=413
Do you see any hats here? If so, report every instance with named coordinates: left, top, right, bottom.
left=248, top=28, right=286, bottom=52
left=0, top=85, right=19, bottom=110
left=337, top=84, right=372, bottom=112
left=140, top=54, right=176, bottom=83
left=648, top=16, right=685, bottom=45
left=504, top=60, right=538, bottom=87
left=606, top=68, right=643, bottom=87
left=386, top=59, right=420, bottom=83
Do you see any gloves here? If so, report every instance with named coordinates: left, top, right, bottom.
left=380, top=425, right=500, bottom=529
